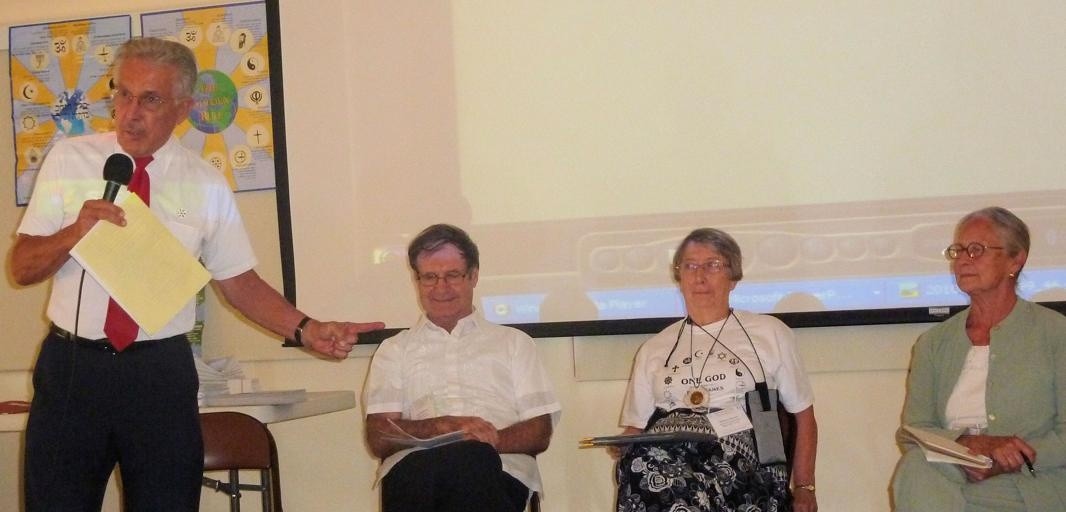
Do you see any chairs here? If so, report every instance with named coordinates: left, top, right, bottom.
left=193, top=412, right=283, bottom=512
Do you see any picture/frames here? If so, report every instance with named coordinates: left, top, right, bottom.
left=136, top=1, right=279, bottom=200
left=8, top=16, right=136, bottom=205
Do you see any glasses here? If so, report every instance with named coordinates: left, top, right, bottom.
left=111, top=88, right=178, bottom=112
left=949, top=242, right=1009, bottom=261
left=417, top=269, right=472, bottom=287
left=672, top=258, right=732, bottom=277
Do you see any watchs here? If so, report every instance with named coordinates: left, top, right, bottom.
left=796, top=484, right=816, bottom=492
left=293, top=316, right=311, bottom=347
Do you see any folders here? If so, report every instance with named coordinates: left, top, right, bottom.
left=578, top=432, right=717, bottom=448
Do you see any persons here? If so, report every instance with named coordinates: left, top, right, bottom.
left=361, top=222, right=562, bottom=512
left=890, top=208, right=1065, bottom=512
left=604, top=228, right=819, bottom=512
left=8, top=37, right=386, bottom=512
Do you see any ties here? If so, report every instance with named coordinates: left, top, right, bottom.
left=103, top=155, right=155, bottom=351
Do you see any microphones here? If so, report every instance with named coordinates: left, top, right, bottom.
left=102, top=153, right=135, bottom=205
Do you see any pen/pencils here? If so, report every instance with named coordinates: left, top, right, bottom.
left=1011, top=435, right=1037, bottom=477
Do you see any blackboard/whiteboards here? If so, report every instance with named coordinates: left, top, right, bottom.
left=266, top=0, right=1066, bottom=348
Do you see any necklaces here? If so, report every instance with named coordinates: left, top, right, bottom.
left=686, top=320, right=726, bottom=410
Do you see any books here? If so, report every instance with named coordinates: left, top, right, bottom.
left=903, top=425, right=993, bottom=470
left=371, top=419, right=464, bottom=491
left=68, top=191, right=213, bottom=339
left=190, top=354, right=305, bottom=407
left=580, top=431, right=700, bottom=449
